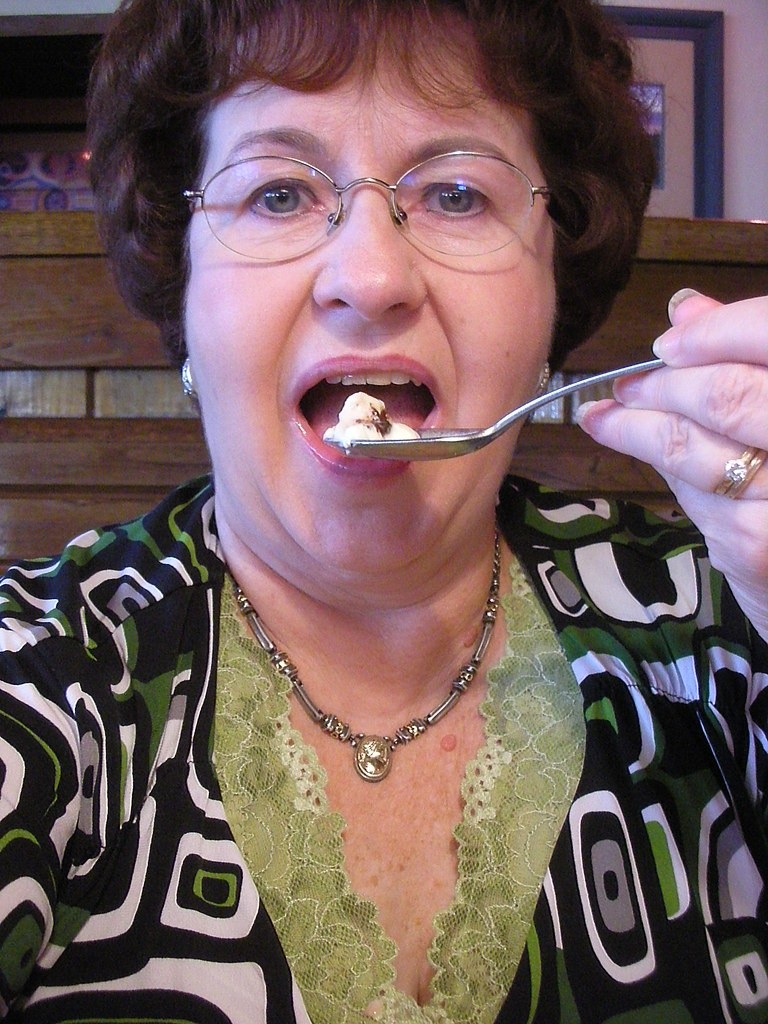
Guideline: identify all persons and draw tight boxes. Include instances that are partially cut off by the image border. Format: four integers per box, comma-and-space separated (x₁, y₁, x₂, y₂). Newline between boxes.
0, 0, 768, 1024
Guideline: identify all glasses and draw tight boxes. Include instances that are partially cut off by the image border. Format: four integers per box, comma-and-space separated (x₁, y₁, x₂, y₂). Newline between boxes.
181, 148, 557, 262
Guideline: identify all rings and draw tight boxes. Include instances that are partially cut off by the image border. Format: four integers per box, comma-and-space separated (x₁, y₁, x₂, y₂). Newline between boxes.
715, 445, 767, 500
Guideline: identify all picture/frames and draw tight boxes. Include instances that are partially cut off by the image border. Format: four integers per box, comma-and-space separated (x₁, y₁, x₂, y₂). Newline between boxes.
595, 4, 724, 223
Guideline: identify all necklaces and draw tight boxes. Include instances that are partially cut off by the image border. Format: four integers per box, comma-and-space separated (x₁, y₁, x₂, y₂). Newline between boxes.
234, 531, 500, 782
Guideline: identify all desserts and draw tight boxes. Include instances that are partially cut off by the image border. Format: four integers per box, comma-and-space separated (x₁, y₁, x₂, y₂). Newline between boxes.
322, 392, 421, 449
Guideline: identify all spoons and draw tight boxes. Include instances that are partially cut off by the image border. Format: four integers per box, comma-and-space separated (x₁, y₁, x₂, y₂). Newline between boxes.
322, 357, 666, 461
